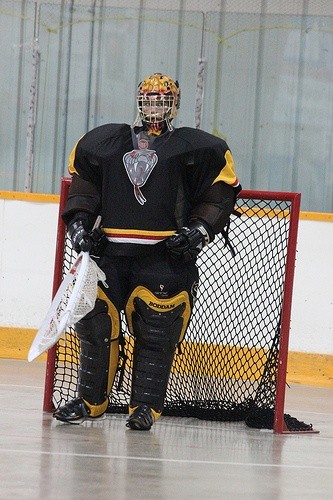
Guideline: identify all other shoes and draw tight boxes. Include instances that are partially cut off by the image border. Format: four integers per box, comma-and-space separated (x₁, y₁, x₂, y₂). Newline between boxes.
53, 397, 106, 425
126, 405, 154, 430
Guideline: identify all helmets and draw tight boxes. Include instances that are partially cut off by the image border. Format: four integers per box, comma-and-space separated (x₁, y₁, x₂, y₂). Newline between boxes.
137, 72, 178, 122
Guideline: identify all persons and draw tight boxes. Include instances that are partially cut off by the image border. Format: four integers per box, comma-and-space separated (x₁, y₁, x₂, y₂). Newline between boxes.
54, 72, 242, 431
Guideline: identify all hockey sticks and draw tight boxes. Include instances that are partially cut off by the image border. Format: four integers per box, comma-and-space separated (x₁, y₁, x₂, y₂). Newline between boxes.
28, 214, 109, 362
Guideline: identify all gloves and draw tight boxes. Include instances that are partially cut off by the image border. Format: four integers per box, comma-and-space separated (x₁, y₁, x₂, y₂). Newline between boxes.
166, 226, 205, 270
66, 216, 108, 259
